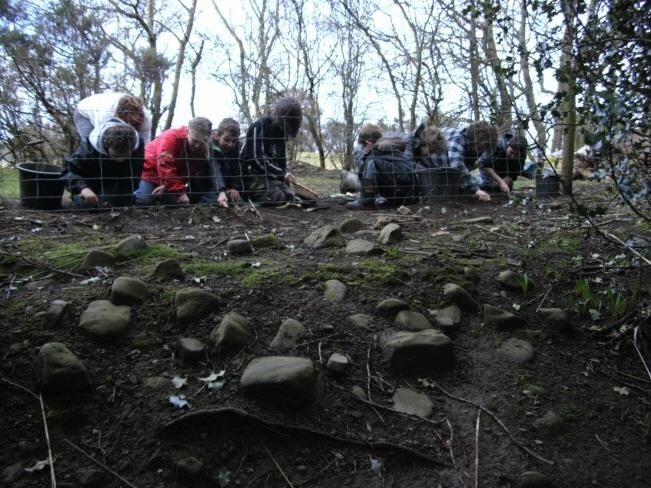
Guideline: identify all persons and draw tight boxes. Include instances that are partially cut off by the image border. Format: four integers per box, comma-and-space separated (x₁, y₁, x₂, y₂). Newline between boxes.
340, 120, 527, 208
63, 91, 303, 207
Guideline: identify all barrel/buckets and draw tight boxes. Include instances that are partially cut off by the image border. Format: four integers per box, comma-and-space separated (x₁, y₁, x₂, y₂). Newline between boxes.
534, 173, 561, 199
15, 161, 67, 210
415, 167, 464, 202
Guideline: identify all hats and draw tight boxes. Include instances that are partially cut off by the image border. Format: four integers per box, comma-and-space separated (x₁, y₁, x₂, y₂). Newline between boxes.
188, 116, 211, 141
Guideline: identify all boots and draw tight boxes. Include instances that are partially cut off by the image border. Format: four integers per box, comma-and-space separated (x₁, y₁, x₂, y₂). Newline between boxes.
347, 178, 376, 210
